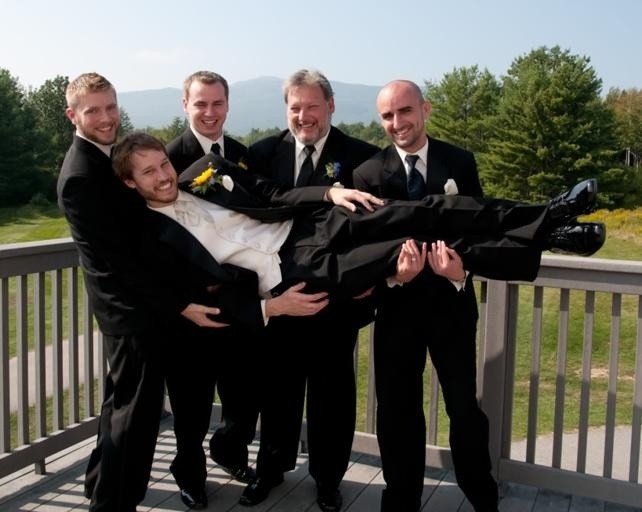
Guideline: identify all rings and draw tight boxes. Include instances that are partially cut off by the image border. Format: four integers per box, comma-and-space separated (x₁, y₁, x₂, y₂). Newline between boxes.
412, 256, 417, 261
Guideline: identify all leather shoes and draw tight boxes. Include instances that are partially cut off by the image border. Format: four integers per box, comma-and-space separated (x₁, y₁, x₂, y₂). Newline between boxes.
181, 486, 207, 509
231, 466, 256, 484
547, 222, 605, 256
551, 179, 598, 217
237, 471, 284, 507
317, 484, 342, 512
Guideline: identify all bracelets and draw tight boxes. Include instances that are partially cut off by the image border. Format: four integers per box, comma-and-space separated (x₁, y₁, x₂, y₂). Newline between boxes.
455, 272, 466, 283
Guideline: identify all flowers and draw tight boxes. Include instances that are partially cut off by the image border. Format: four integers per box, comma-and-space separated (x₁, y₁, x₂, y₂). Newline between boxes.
323, 163, 343, 180
188, 167, 223, 195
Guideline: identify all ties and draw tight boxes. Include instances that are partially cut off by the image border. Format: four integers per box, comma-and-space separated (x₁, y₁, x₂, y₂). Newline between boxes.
296, 146, 316, 186
211, 144, 220, 157
405, 155, 426, 199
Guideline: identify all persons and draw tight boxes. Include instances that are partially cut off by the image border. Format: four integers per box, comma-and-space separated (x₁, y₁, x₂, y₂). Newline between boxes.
237, 67, 398, 510
155, 72, 256, 510
111, 130, 607, 337
56, 72, 231, 510
350, 81, 501, 509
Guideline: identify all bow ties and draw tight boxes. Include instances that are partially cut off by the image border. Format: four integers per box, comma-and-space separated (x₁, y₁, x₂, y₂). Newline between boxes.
175, 202, 199, 226
